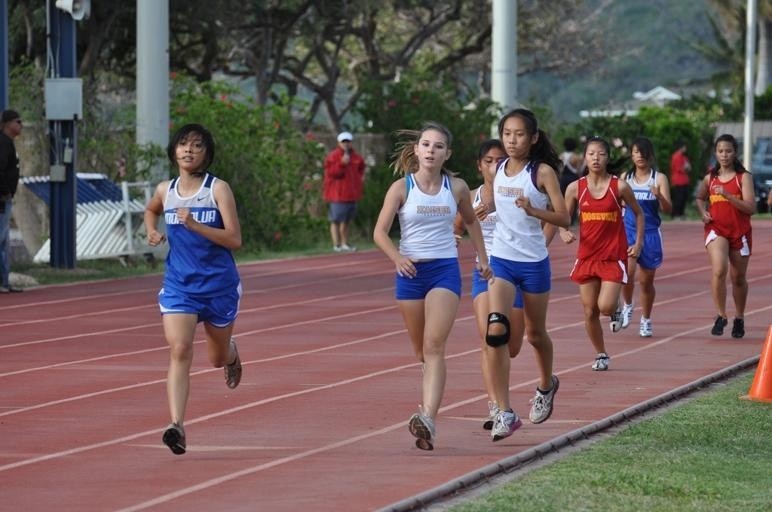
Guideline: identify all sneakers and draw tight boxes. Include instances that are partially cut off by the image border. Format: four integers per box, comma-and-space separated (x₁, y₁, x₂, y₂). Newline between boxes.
711, 314, 727, 335
640, 315, 652, 337
609, 303, 623, 332
408, 413, 436, 450
162, 423, 186, 454
333, 246, 342, 252
622, 304, 632, 328
342, 243, 357, 251
732, 319, 745, 338
483, 401, 499, 430
224, 338, 241, 389
592, 352, 609, 371
491, 409, 523, 441
529, 374, 559, 424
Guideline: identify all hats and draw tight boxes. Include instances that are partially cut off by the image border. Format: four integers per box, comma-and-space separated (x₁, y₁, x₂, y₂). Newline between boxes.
336, 131, 352, 142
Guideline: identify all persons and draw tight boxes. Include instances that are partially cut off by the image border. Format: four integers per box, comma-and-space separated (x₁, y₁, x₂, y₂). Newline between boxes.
556, 138, 582, 227
695, 134, 758, 338
474, 108, 571, 440
557, 138, 645, 370
619, 138, 671, 336
452, 138, 525, 431
143, 122, 242, 454
0, 109, 23, 293
322, 132, 366, 252
372, 120, 493, 451
668, 142, 692, 219
